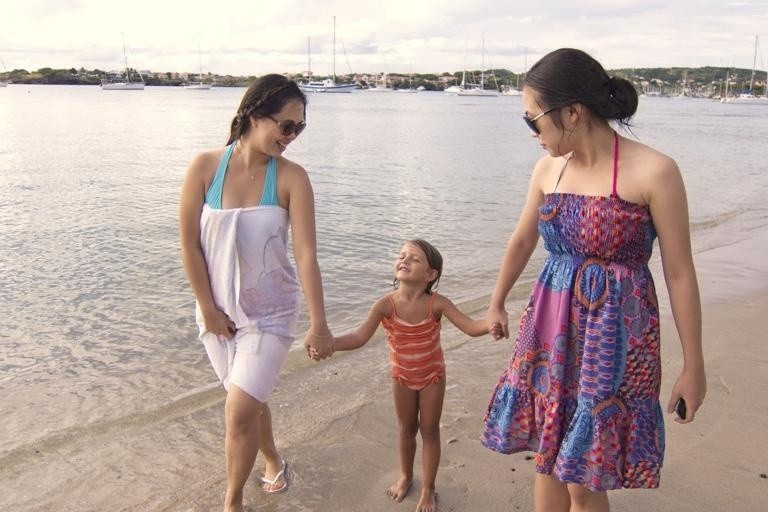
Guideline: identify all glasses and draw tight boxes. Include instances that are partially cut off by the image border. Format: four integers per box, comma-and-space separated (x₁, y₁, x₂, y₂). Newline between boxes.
267, 114, 306, 137
522, 100, 584, 135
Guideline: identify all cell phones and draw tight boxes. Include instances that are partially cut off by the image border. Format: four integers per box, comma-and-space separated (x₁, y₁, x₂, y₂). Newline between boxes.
675, 398, 687, 419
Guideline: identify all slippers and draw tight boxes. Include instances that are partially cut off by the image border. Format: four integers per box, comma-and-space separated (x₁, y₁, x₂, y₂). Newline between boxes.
260, 459, 287, 494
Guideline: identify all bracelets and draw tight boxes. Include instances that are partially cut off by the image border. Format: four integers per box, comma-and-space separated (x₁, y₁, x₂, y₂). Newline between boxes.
313, 330, 333, 340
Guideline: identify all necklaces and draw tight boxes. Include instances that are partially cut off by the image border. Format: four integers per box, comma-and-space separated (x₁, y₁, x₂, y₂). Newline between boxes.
235, 145, 264, 184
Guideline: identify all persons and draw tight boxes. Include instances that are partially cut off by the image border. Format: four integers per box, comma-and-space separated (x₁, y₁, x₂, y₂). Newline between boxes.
311, 237, 503, 511
177, 74, 339, 511
481, 46, 708, 512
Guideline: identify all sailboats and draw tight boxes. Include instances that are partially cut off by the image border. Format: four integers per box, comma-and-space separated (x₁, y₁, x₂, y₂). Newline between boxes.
296, 14, 525, 97
180, 61, 213, 90
641, 32, 766, 105
98, 44, 145, 91
